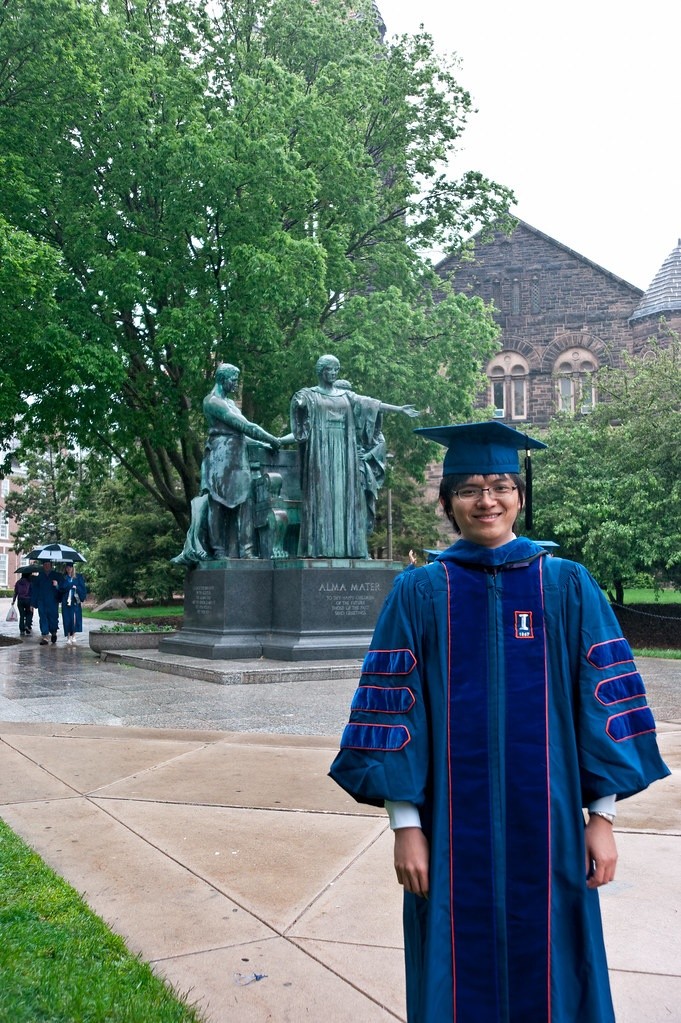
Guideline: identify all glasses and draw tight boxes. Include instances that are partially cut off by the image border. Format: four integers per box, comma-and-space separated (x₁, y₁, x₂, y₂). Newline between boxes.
449, 485, 519, 503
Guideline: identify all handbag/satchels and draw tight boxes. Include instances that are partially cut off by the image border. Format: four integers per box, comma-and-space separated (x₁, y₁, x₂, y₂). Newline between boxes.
6, 606, 18, 622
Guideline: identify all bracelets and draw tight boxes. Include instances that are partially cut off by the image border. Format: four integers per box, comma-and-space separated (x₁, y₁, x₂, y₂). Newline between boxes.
595, 812, 615, 825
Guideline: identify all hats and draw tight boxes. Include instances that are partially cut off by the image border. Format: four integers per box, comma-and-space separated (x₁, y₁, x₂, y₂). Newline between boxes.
65, 562, 77, 567
412, 421, 547, 531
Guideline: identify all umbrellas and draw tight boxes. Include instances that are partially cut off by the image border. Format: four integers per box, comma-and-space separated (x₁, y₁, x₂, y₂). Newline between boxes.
25, 543, 87, 562
14, 565, 42, 573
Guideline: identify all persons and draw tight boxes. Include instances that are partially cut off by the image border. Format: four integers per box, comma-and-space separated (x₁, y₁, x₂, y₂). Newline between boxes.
28, 559, 64, 645
531, 541, 560, 557
11, 573, 33, 635
62, 562, 86, 644
290, 355, 420, 559
327, 421, 672, 1023
393, 549, 443, 587
278, 379, 386, 532
170, 362, 281, 565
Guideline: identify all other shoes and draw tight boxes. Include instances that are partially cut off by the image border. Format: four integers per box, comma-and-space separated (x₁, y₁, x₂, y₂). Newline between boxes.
51, 636, 57, 643
40, 639, 48, 645
71, 635, 76, 642
66, 640, 73, 646
24, 626, 31, 634
20, 631, 24, 634
